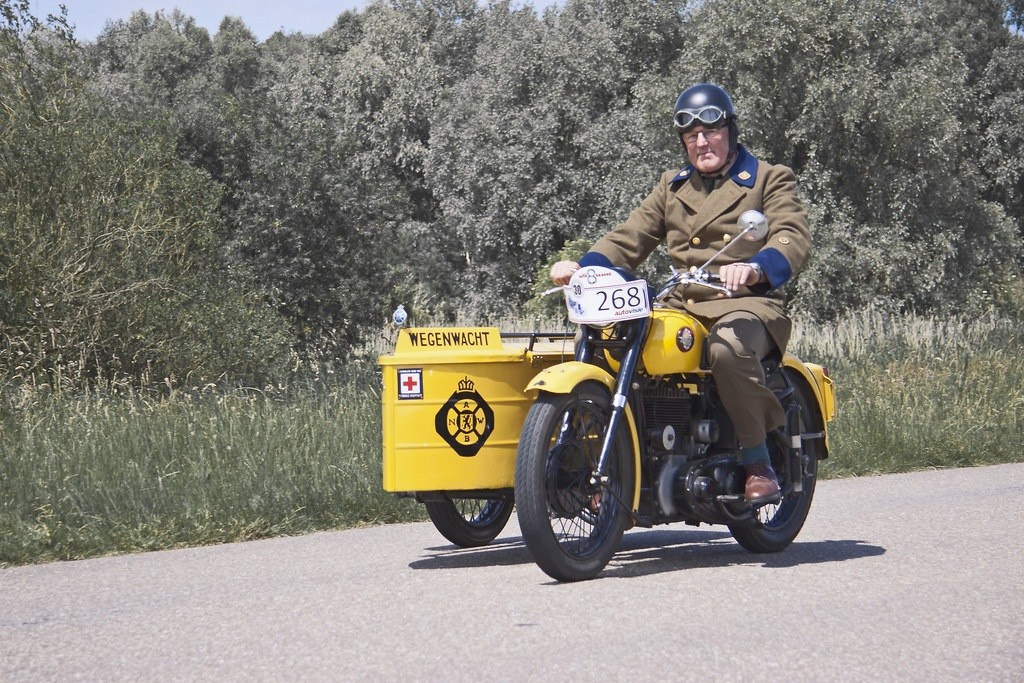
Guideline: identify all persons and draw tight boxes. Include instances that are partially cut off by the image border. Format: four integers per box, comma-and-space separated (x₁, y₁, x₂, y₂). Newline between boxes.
550, 84, 815, 504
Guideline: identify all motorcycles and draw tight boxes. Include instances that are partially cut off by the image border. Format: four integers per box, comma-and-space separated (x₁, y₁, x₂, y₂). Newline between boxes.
382, 209, 837, 582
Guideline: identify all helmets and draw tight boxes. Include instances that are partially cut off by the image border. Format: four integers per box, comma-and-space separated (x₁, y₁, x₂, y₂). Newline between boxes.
673, 83, 735, 127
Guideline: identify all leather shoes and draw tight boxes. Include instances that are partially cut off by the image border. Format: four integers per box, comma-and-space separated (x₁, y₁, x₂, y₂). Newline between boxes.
743, 463, 781, 504
591, 493, 602, 512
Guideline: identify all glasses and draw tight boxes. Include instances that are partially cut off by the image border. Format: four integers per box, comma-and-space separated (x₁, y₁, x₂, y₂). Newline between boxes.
673, 105, 727, 129
